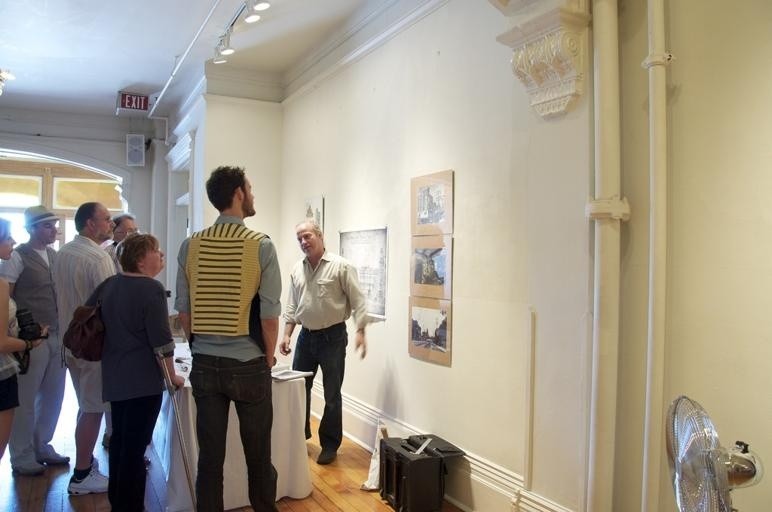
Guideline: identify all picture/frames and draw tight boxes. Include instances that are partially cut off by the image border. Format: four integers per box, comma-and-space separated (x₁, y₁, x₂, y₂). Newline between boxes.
411, 236, 453, 300
408, 297, 452, 367
339, 226, 388, 319
411, 170, 454, 235
303, 195, 325, 238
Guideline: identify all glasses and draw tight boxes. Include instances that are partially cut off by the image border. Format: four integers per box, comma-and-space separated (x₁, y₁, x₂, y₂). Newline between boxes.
117, 231, 134, 236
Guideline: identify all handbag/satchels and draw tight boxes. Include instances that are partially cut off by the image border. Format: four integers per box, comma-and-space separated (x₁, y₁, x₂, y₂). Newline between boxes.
60, 274, 110, 370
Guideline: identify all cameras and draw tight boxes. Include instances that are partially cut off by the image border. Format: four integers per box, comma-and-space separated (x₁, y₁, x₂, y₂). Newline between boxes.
15, 307, 42, 342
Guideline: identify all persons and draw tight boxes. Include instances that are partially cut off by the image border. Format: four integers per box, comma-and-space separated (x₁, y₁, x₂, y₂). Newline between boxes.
1, 205, 71, 475
0, 217, 50, 459
102, 214, 139, 448
51, 203, 116, 497
174, 166, 282, 512
84, 234, 185, 512
279, 217, 368, 463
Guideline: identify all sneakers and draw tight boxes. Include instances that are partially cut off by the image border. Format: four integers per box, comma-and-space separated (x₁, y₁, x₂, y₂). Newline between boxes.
37, 446, 71, 465
66, 468, 109, 496
10, 457, 46, 475
316, 447, 338, 464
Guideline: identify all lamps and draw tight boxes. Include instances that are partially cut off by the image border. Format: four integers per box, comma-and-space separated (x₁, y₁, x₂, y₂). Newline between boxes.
252, 0, 270, 12
245, 2, 261, 23
213, 50, 227, 64
221, 34, 236, 55
0, 67, 14, 101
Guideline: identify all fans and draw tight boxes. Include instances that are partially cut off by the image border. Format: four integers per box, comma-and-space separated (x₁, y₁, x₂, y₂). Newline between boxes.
664, 396, 765, 507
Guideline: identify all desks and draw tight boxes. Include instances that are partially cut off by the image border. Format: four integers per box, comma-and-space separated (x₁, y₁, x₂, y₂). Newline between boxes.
152, 343, 314, 512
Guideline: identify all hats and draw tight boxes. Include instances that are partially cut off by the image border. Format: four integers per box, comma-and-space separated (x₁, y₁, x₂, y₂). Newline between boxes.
24, 205, 61, 228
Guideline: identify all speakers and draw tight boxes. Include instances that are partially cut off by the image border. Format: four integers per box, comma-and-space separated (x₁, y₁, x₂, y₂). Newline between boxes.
125, 133, 146, 167
